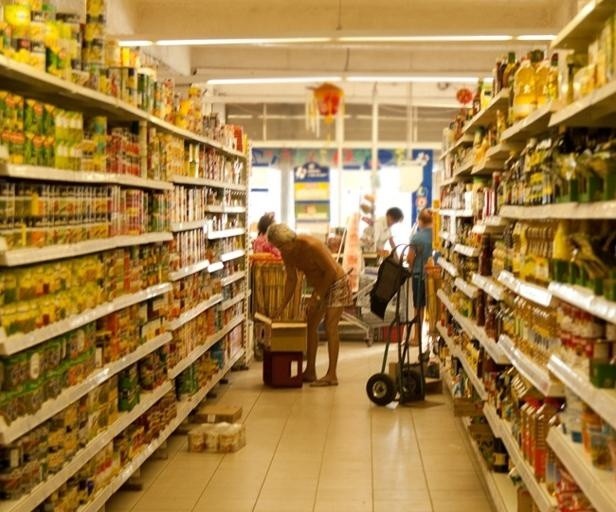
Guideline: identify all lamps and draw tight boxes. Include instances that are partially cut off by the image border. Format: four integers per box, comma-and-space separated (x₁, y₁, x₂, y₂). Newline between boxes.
426, 29, 554, 45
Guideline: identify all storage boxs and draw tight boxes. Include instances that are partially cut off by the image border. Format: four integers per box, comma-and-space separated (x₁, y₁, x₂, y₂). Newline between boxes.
196, 404, 242, 422
187, 422, 246, 453
254, 312, 310, 356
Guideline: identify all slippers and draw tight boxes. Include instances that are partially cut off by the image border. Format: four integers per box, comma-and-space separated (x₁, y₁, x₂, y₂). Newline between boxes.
292, 372, 338, 387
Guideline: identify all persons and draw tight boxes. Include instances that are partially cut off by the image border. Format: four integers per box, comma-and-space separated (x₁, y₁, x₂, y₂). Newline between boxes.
252, 214, 282, 256
376, 206, 413, 269
324, 224, 383, 263
267, 223, 354, 388
402, 209, 433, 346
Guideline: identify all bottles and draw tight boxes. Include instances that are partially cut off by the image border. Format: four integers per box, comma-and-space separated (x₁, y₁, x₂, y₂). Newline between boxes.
517, 396, 564, 496
508, 54, 540, 120
477, 136, 556, 286
474, 287, 616, 392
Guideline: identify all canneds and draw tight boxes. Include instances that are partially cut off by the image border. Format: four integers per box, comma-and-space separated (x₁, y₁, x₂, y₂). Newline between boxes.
189, 422, 245, 452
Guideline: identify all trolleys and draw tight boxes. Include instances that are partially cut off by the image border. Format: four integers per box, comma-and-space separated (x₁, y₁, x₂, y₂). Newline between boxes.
366, 245, 425, 405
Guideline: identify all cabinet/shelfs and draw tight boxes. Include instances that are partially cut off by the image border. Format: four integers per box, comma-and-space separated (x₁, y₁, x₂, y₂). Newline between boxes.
429, 92, 531, 392
162, 121, 250, 406
484, 1, 615, 512
0, 52, 190, 510
